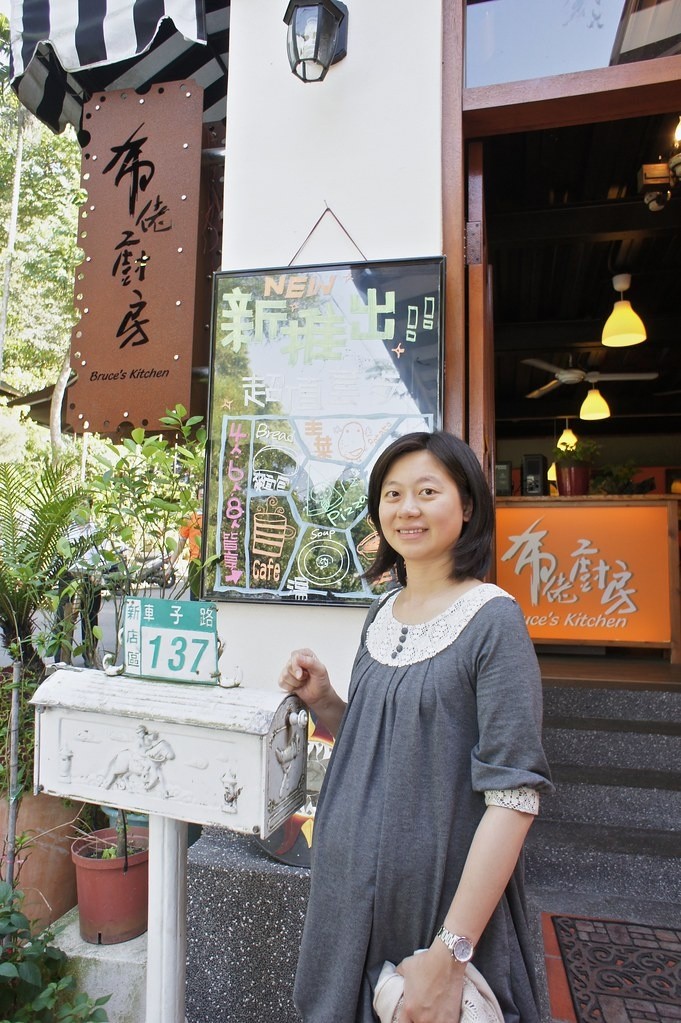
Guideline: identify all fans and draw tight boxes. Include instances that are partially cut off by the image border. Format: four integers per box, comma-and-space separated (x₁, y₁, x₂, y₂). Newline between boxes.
518, 355, 659, 399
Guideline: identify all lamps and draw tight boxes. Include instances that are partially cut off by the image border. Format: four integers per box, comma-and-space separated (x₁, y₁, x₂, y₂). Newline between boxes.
556, 419, 578, 450
580, 383, 611, 420
601, 273, 647, 348
282, 0, 349, 83
547, 459, 557, 480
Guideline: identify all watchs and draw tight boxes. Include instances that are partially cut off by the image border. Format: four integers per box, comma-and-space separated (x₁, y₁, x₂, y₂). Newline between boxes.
437, 927, 475, 962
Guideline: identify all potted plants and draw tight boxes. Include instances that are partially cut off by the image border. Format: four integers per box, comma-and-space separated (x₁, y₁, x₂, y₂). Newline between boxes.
0, 404, 221, 946
552, 438, 601, 496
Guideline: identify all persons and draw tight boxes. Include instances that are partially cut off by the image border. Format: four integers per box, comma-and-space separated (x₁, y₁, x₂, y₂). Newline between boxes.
277, 430, 554, 1023
